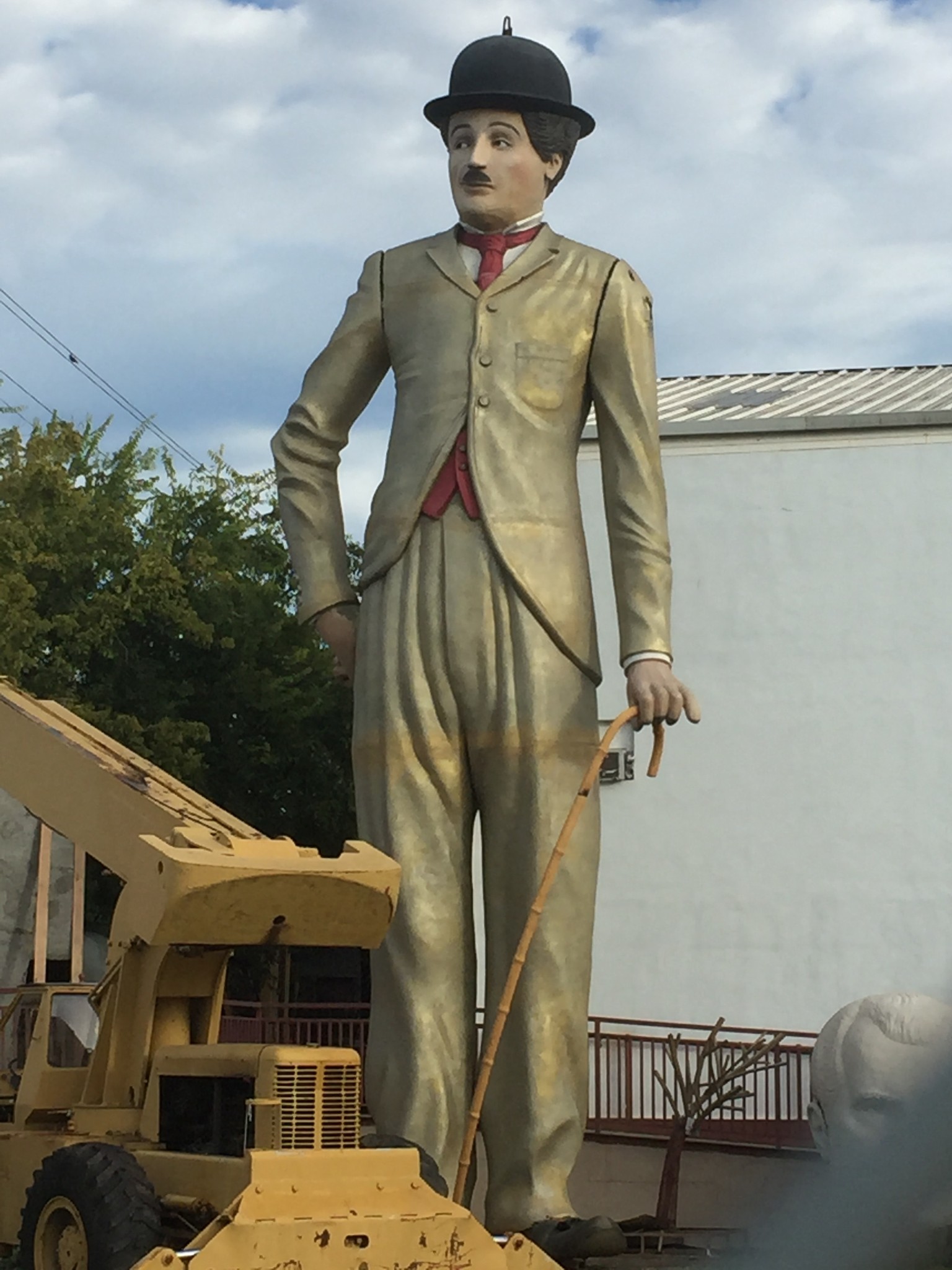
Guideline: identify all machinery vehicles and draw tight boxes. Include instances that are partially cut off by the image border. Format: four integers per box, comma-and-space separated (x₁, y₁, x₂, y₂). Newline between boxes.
0, 672, 568, 1270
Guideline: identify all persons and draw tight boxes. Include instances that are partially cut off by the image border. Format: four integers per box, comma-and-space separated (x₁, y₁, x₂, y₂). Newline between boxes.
807, 994, 951, 1270
270, 15, 702, 1260
